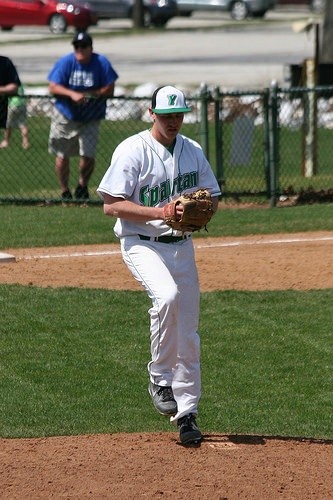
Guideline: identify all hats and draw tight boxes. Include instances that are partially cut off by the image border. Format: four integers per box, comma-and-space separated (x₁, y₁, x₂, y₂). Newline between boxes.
151, 84, 192, 114
72, 32, 92, 44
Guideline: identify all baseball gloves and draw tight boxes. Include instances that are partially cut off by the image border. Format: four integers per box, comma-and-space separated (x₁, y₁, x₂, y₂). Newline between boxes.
164, 189, 213, 233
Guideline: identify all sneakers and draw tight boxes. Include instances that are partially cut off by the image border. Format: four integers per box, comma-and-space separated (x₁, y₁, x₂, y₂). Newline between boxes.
147, 381, 178, 415
176, 413, 203, 445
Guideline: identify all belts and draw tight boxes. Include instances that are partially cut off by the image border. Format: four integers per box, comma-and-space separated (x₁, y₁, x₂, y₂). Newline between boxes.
137, 234, 192, 244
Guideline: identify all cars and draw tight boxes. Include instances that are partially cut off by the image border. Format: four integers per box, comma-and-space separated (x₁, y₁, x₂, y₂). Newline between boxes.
82, 0, 175, 30
177, 1, 271, 22
0, 1, 98, 35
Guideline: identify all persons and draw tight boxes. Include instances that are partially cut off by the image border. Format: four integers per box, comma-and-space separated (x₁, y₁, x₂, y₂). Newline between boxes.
0, 81, 32, 151
0, 56, 21, 130
96, 86, 223, 445
47, 32, 118, 200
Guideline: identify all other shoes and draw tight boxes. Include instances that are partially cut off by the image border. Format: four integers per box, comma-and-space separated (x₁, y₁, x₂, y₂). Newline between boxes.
0, 139, 9, 149
61, 187, 73, 200
21, 140, 31, 150
74, 184, 89, 200
